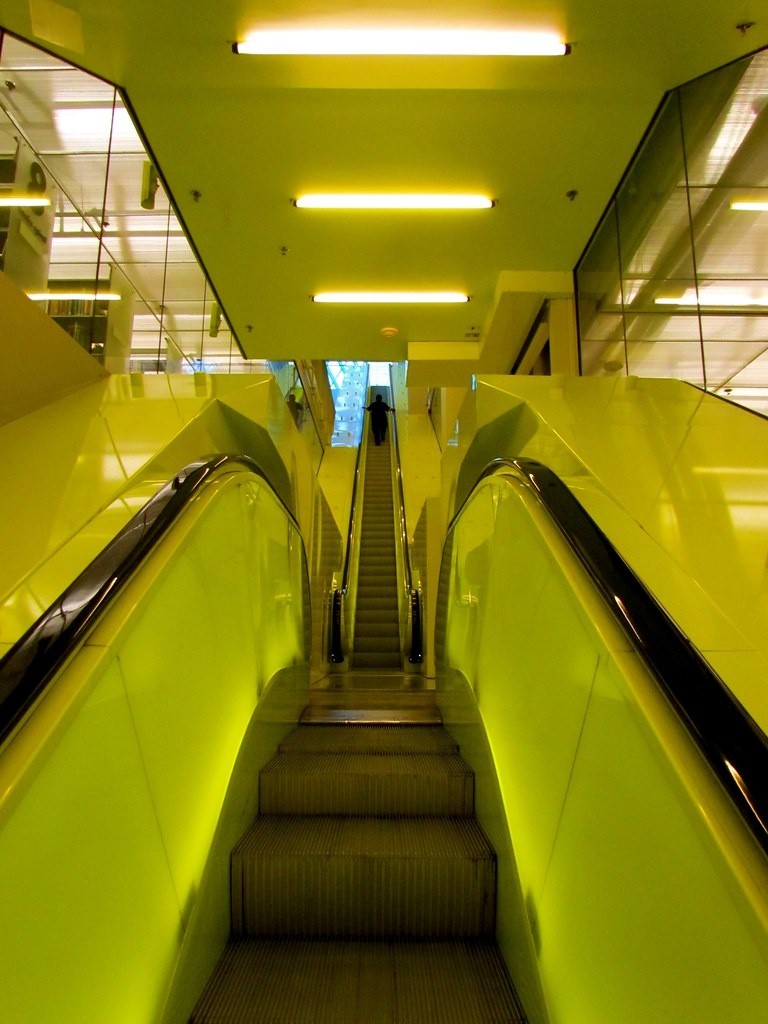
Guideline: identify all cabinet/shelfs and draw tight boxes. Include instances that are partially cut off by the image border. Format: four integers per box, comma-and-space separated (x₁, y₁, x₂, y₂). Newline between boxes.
47, 262, 133, 377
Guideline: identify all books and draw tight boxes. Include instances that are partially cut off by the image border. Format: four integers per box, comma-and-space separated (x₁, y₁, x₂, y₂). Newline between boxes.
48, 294, 107, 356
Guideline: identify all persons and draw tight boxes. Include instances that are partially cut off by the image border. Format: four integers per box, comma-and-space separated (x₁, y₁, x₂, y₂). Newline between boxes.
286, 393, 304, 425
362, 394, 396, 446
